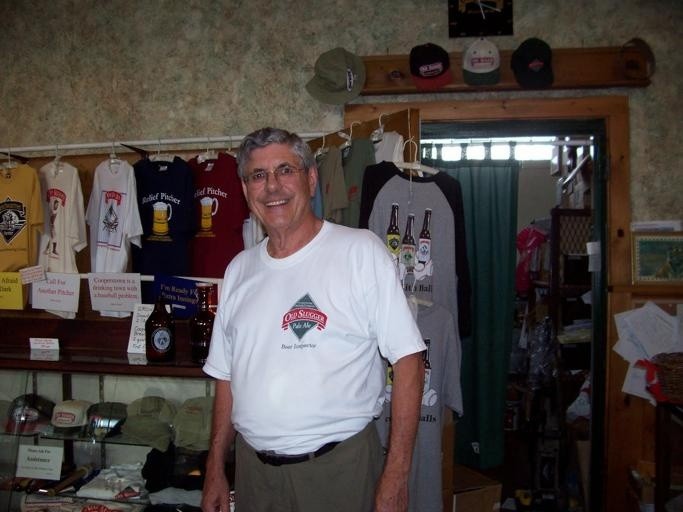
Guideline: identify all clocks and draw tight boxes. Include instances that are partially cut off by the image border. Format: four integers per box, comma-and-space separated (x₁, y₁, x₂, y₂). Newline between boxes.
447, 1, 515, 39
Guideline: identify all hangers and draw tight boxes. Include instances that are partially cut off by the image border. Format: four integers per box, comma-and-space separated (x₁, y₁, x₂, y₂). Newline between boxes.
1, 148, 36, 173
366, 139, 457, 184
339, 120, 371, 151
372, 113, 402, 146
137, 138, 188, 169
94, 140, 133, 169
310, 129, 339, 157
192, 136, 232, 170
40, 146, 79, 175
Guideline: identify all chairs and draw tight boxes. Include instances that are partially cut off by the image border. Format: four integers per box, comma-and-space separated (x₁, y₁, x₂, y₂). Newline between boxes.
529, 205, 592, 330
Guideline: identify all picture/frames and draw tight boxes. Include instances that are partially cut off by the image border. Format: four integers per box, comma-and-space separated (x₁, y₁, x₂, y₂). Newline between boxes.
632, 231, 683, 286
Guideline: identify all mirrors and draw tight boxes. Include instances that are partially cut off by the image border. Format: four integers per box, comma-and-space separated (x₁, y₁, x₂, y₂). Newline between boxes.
419, 115, 606, 512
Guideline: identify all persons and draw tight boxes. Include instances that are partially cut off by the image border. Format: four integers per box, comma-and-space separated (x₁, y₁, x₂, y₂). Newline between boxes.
41, 202, 63, 255
201, 127, 428, 512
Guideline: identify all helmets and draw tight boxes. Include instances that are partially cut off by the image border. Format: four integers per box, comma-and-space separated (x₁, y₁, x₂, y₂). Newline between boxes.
612, 38, 655, 79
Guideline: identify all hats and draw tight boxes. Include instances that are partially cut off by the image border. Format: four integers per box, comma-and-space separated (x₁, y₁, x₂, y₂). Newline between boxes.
51, 400, 93, 435
410, 44, 454, 92
305, 48, 366, 105
80, 402, 128, 441
510, 37, 556, 91
462, 37, 500, 87
6, 394, 55, 433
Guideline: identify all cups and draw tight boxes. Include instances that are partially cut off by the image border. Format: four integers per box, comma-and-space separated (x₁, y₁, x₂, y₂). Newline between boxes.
200, 196, 219, 230
154, 202, 171, 234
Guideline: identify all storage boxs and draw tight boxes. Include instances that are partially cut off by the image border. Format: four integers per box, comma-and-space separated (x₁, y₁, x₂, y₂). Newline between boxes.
453, 463, 501, 511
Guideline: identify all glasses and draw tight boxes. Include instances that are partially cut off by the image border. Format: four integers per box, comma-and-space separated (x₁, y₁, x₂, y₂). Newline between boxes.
244, 166, 310, 183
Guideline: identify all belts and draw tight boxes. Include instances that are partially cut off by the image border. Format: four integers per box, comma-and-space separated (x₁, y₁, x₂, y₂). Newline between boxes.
254, 439, 341, 466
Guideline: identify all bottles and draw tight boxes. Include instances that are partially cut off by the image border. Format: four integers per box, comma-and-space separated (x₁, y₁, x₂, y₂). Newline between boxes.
416, 208, 433, 264
386, 203, 402, 259
400, 212, 416, 273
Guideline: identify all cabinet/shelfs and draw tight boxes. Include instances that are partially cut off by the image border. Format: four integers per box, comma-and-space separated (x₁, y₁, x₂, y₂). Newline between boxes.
0, 307, 239, 512
